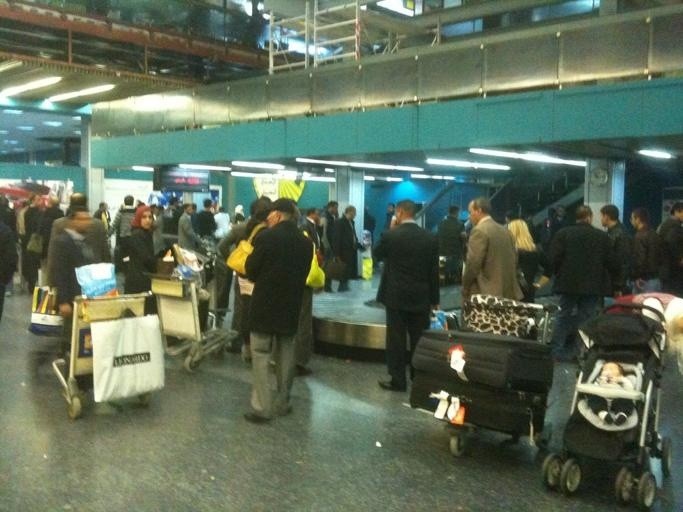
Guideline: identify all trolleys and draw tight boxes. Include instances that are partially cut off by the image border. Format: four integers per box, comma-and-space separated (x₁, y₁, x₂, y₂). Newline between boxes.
143, 271, 239, 373
52, 290, 154, 417
448, 292, 561, 456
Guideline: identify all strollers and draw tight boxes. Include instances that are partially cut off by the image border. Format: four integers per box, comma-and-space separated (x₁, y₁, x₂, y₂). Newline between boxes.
539, 302, 672, 510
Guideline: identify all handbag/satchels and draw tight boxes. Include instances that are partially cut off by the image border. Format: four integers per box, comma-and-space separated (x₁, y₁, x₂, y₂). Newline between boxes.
326, 256, 347, 280
305, 242, 326, 289
429, 310, 459, 330
26, 234, 42, 253
28, 286, 64, 336
89, 308, 166, 406
225, 222, 267, 278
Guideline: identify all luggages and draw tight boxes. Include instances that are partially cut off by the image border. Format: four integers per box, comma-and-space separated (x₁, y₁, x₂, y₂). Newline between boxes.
408, 371, 548, 434
409, 327, 554, 391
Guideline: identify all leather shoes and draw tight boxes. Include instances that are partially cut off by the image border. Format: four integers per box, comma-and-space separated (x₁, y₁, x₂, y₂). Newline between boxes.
376, 378, 408, 392
225, 342, 313, 424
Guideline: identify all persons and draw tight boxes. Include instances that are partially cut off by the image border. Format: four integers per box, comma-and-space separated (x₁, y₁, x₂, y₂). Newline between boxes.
438, 196, 539, 302
299, 201, 366, 292
1, 190, 271, 363
373, 195, 442, 391
588, 356, 635, 424
243, 196, 316, 422
383, 203, 395, 229
538, 202, 681, 361
